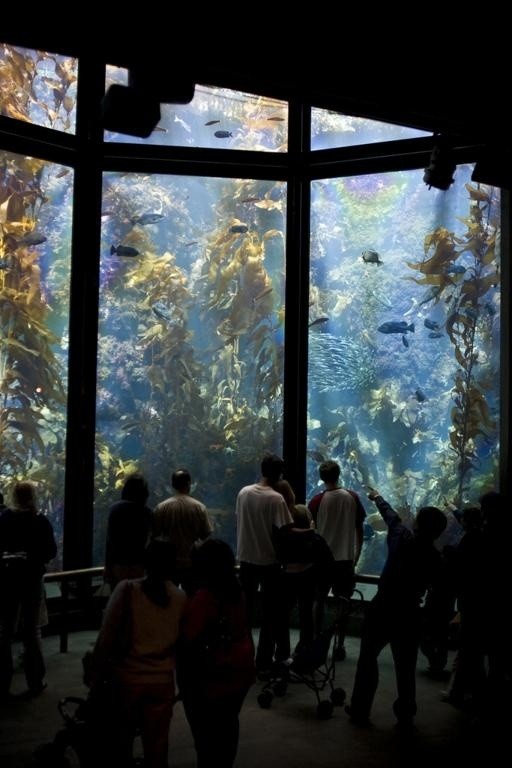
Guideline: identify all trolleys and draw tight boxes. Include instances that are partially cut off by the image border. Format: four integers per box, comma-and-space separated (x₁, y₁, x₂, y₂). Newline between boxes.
255, 588, 365, 719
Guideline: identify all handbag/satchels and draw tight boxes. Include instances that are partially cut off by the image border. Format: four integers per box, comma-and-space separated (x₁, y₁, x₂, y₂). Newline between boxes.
109, 629, 132, 664
